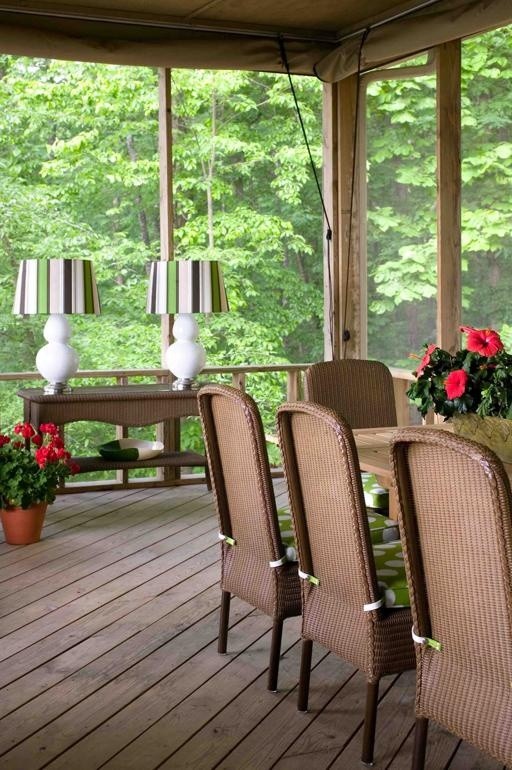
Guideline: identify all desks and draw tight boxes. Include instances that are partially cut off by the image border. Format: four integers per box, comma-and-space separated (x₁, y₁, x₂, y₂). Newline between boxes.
14, 384, 210, 491
267, 421, 454, 523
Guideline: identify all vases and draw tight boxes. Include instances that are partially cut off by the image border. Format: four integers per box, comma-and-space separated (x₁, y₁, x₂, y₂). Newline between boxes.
1, 506, 45, 546
452, 415, 512, 466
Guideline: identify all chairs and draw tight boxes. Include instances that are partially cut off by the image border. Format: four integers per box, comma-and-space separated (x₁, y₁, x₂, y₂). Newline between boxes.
277, 403, 416, 762
388, 426, 512, 770
197, 384, 399, 693
306, 359, 399, 507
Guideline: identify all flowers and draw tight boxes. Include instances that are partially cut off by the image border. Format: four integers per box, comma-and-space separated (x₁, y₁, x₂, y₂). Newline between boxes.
0, 424, 80, 507
407, 324, 512, 422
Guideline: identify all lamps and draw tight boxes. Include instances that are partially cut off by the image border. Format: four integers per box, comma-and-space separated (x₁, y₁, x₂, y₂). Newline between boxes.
147, 259, 230, 391
10, 256, 101, 392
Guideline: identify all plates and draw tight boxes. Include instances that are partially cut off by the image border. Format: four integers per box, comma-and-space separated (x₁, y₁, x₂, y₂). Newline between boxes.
98, 438, 165, 462
